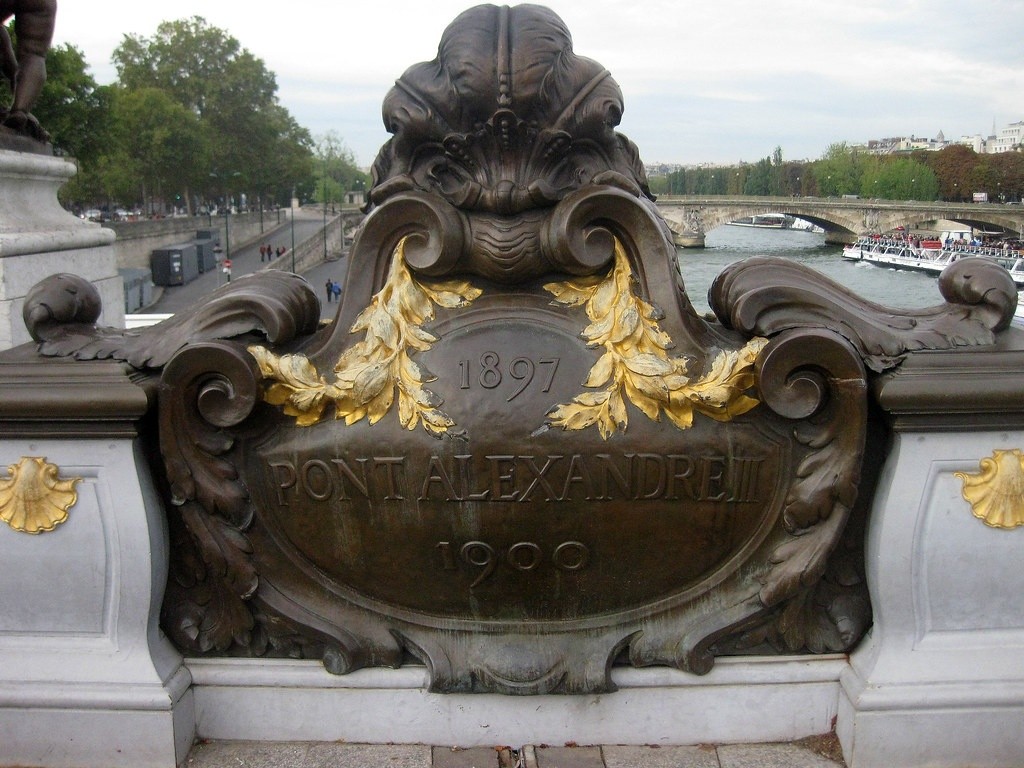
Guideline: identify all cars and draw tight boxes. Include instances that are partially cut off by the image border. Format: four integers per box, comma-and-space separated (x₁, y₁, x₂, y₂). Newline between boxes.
84, 209, 101, 222
1006, 202, 1019, 206
112, 208, 134, 221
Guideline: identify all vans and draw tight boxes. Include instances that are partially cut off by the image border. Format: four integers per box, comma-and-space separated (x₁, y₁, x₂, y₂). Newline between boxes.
841, 195, 861, 200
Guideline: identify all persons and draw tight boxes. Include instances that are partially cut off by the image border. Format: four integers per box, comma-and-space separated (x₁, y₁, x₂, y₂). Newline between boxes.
333, 282, 342, 303
326, 279, 333, 303
793, 193, 801, 197
869, 233, 1022, 259
260, 243, 286, 262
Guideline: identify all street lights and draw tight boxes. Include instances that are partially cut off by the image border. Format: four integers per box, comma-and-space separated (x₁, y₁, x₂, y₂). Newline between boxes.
209, 173, 243, 284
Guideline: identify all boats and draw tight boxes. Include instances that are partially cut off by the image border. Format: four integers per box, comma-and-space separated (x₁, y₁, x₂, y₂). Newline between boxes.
725, 213, 796, 229
841, 230, 1024, 290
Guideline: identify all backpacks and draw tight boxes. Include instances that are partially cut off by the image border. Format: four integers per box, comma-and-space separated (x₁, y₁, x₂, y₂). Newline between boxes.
327, 283, 332, 289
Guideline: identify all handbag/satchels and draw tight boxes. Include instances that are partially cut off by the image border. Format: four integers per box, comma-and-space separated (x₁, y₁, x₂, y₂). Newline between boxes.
338, 287, 341, 293
269, 251, 273, 254
